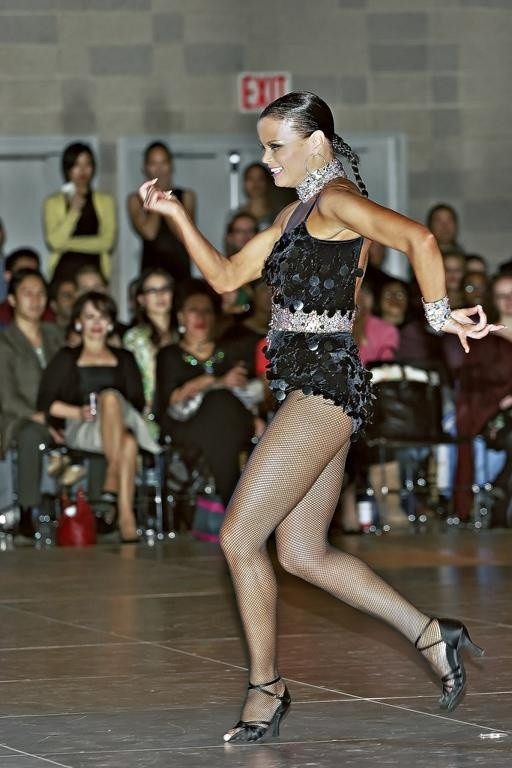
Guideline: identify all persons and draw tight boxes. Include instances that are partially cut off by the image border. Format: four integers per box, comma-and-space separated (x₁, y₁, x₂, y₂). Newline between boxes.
134, 91, 512, 742
1, 141, 511, 550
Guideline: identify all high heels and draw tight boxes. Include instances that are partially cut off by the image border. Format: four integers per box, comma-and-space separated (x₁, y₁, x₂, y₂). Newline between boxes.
414, 617, 485, 712
223, 675, 291, 744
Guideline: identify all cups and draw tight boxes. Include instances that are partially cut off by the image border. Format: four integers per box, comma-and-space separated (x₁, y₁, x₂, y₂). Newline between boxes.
60, 180, 76, 201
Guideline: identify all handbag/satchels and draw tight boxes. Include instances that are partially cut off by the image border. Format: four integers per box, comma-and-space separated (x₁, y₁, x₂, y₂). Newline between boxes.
191, 478, 225, 542
366, 345, 442, 441
55, 489, 98, 545
